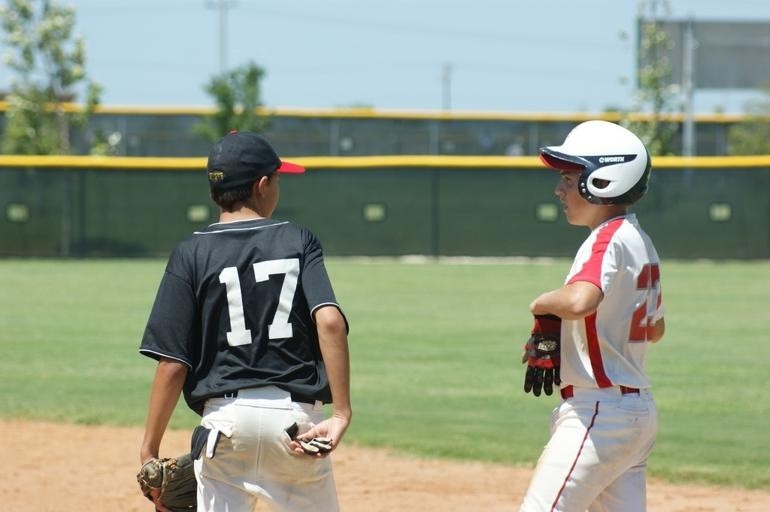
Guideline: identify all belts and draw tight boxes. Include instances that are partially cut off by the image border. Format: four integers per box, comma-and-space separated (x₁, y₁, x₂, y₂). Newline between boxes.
209, 389, 317, 406
559, 383, 641, 400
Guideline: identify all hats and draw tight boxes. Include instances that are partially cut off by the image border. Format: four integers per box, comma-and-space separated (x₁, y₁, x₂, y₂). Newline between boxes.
204, 128, 309, 191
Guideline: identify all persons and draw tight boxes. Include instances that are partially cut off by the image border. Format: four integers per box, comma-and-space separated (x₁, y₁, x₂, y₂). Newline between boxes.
135, 128, 353, 512
515, 118, 666, 512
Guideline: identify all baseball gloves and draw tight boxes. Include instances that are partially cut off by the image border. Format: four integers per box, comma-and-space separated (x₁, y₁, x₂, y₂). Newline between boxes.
139, 454, 197, 512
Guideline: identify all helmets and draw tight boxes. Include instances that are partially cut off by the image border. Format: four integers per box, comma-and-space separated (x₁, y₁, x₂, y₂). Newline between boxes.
536, 118, 654, 208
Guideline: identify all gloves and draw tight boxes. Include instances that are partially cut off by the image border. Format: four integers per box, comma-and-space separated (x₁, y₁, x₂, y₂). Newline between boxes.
286, 421, 334, 459
520, 312, 563, 398
189, 425, 235, 463
